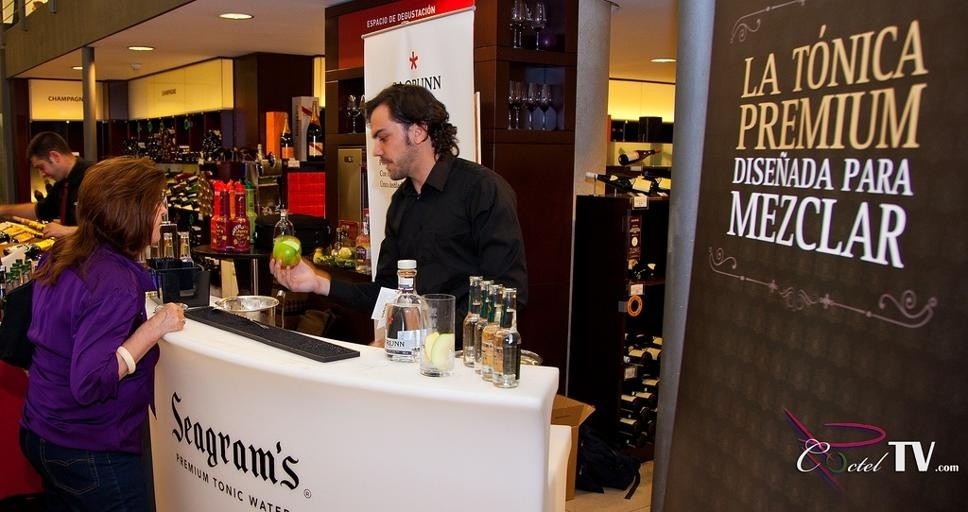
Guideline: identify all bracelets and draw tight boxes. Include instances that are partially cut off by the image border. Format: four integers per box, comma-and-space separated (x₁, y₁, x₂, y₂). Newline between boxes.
116, 346, 137, 374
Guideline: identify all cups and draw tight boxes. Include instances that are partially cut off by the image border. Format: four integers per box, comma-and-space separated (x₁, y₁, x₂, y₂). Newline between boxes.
420, 294, 457, 378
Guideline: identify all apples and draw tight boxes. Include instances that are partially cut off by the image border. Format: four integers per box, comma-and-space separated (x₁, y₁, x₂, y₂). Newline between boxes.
272, 234, 303, 268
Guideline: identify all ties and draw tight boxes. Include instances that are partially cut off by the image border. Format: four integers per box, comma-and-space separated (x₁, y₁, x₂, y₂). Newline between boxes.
60, 181, 68, 225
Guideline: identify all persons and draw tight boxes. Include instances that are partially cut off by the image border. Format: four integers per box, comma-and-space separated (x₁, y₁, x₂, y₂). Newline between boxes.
0, 131, 96, 240
19, 155, 186, 512
268, 82, 529, 353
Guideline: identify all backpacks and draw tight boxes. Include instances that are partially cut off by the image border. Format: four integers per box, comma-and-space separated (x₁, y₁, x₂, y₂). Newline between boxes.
580, 426, 641, 499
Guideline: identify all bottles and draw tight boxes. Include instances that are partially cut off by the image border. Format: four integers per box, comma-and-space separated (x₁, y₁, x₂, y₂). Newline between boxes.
136, 171, 257, 268
463, 275, 521, 388
620, 332, 662, 449
330, 207, 371, 276
280, 113, 295, 165
124, 114, 274, 177
384, 260, 421, 364
511, 0, 548, 52
508, 80, 552, 131
629, 262, 657, 281
585, 147, 671, 195
345, 94, 364, 134
0, 215, 55, 324
273, 209, 295, 250
307, 101, 324, 162
